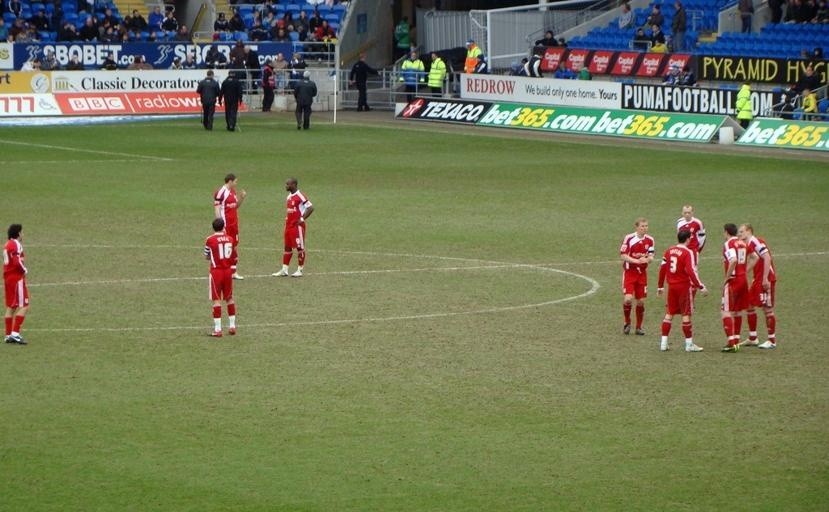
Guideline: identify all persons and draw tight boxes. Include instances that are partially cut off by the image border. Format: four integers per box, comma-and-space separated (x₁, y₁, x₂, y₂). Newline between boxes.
635, 26, 669, 54
261, 58, 278, 113
428, 53, 447, 98
510, 48, 544, 78
1, 223, 32, 346
272, 176, 315, 278
348, 53, 382, 113
203, 217, 238, 337
77, 5, 193, 43
213, 173, 246, 280
781, 47, 826, 119
619, 4, 637, 29
619, 218, 656, 335
129, 54, 154, 70
272, 52, 308, 93
737, 223, 777, 349
292, 70, 318, 130
206, 41, 262, 93
719, 222, 760, 353
100, 53, 119, 70
672, 2, 687, 50
645, 4, 664, 28
399, 52, 426, 103
536, 30, 567, 48
767, 1, 829, 26
556, 61, 578, 79
21, 48, 88, 72
197, 70, 221, 132
738, 0, 754, 35
213, 5, 338, 42
735, 81, 754, 128
222, 70, 243, 132
463, 39, 486, 74
663, 65, 697, 88
1, 0, 79, 43
170, 54, 198, 70
656, 228, 708, 353
675, 204, 707, 312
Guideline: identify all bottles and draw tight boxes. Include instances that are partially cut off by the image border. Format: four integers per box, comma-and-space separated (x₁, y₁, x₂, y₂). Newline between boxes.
466, 39, 474, 45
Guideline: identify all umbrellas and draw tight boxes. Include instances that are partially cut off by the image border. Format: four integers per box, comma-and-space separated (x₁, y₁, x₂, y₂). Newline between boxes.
204, 125, 207, 130
229, 127, 234, 131
304, 127, 310, 130
226, 126, 229, 130
208, 127, 212, 130
297, 125, 302, 129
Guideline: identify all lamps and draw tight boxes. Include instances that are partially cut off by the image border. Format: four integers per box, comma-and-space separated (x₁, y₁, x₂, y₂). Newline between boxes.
688, 23, 828, 60
238, 4, 345, 31
0, 1, 123, 40
565, 1, 738, 54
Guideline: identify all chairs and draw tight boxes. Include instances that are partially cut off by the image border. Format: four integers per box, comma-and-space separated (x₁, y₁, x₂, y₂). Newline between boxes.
232, 274, 244, 280
4, 337, 11, 343
685, 344, 703, 352
229, 327, 235, 335
623, 325, 630, 335
661, 343, 669, 351
8, 335, 26, 344
740, 339, 759, 346
636, 329, 644, 334
209, 331, 222, 337
735, 344, 740, 351
721, 345, 737, 353
291, 271, 303, 277
758, 341, 776, 349
272, 270, 289, 276
